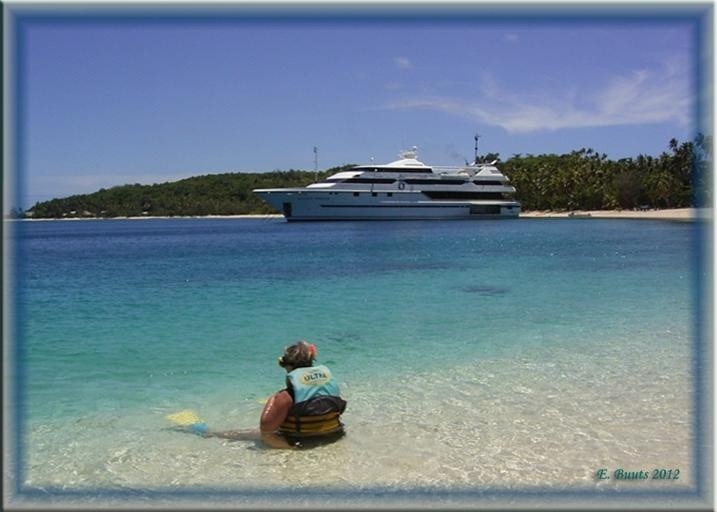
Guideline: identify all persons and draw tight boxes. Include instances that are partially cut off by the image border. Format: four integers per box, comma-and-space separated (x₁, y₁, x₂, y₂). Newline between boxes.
167, 341, 348, 450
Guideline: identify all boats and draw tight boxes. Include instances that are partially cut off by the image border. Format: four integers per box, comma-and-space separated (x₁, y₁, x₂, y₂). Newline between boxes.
251, 147, 523, 224
